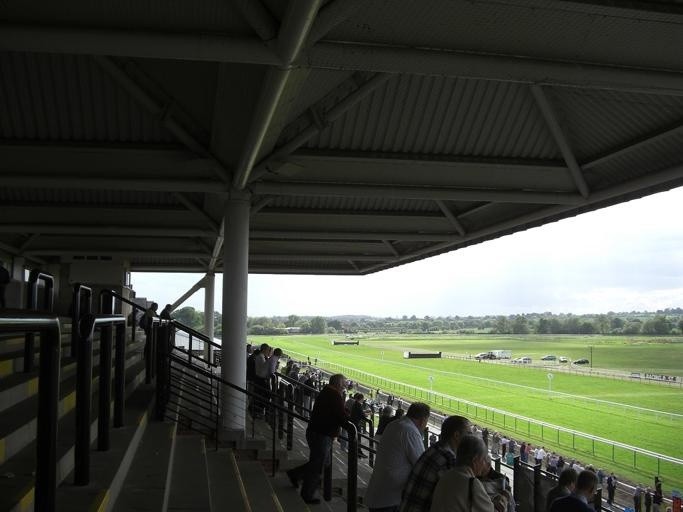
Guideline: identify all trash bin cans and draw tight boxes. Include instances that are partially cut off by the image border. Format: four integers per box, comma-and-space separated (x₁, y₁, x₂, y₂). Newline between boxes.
506, 453, 513, 464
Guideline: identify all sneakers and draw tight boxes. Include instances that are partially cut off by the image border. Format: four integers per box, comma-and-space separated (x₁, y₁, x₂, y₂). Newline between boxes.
303, 496, 320, 504
358, 451, 367, 458
286, 469, 299, 488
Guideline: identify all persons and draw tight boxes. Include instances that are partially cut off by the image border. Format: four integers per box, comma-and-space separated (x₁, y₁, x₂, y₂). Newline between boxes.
139, 301, 158, 360
286, 374, 405, 505
127, 309, 140, 327
360, 402, 666, 511
161, 304, 174, 320
246, 343, 325, 423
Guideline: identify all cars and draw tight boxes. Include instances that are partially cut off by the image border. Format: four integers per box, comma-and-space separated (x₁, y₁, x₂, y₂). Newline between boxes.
511, 356, 533, 363
573, 359, 589, 364
541, 355, 557, 360
474, 353, 489, 359
559, 356, 568, 362
